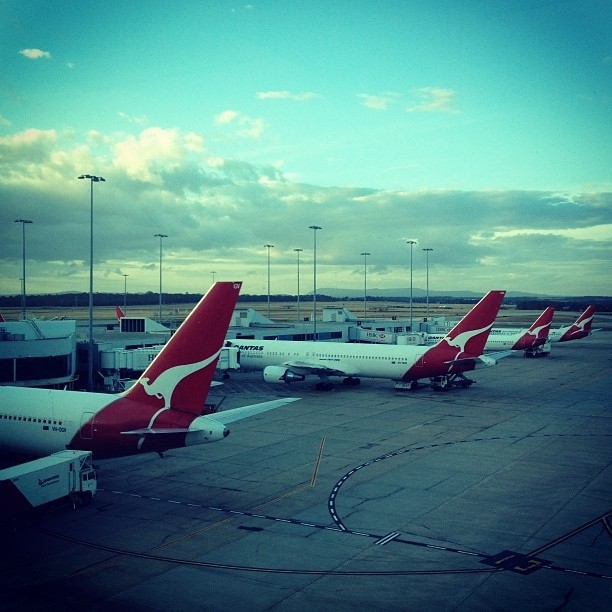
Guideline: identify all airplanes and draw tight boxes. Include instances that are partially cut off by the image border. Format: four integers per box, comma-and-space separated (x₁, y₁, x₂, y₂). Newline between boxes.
423, 304, 555, 360
490, 302, 596, 343
0, 281, 243, 468
225, 290, 507, 398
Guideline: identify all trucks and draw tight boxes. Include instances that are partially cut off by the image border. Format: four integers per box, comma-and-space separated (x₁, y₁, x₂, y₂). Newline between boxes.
0, 449, 97, 514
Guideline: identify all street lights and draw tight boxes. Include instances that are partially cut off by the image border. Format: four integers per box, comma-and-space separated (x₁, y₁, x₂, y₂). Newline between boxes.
294, 248, 304, 322
405, 238, 419, 333
263, 244, 275, 320
152, 234, 169, 328
13, 218, 34, 318
308, 225, 321, 341
77, 174, 106, 390
422, 248, 434, 317
360, 252, 371, 323
